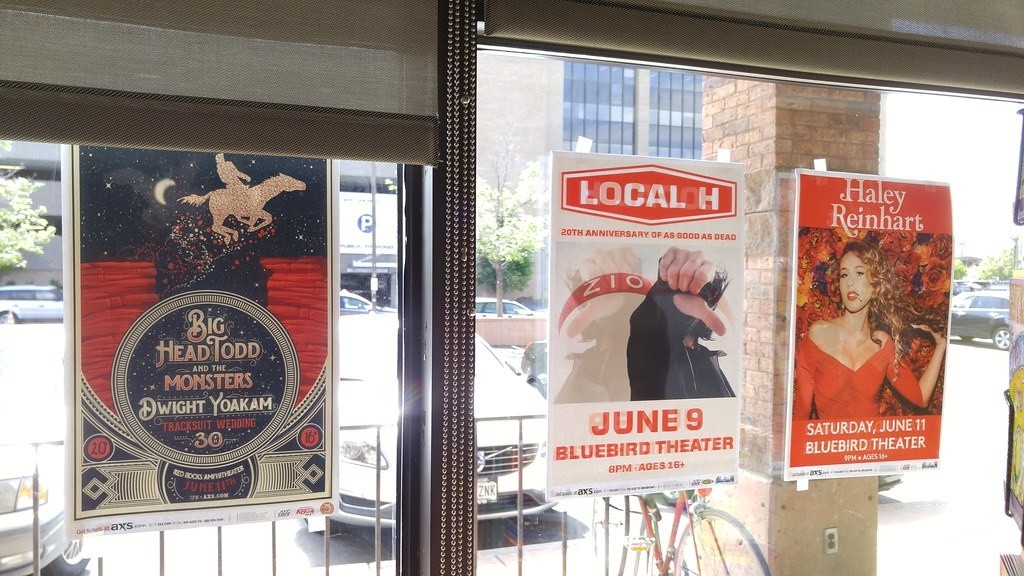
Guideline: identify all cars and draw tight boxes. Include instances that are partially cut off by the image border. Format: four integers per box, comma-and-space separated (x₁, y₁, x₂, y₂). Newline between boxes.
0, 283, 95, 576
336, 289, 560, 528
949, 279, 1012, 351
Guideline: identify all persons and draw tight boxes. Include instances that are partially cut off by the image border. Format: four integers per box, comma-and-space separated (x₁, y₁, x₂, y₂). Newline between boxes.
627, 246, 736, 402
793, 235, 946, 421
